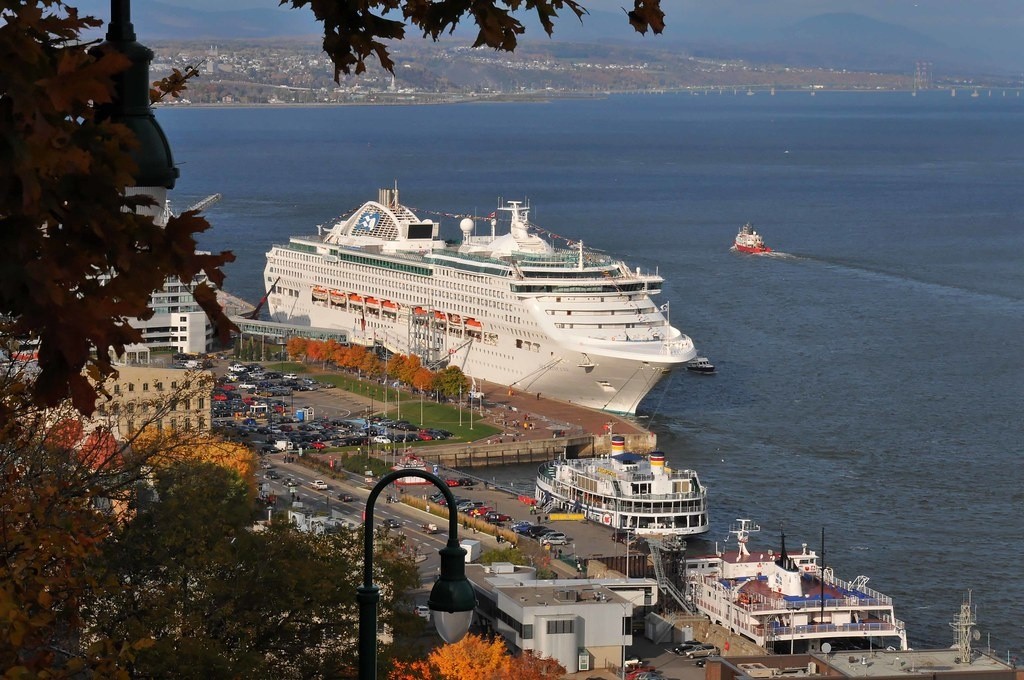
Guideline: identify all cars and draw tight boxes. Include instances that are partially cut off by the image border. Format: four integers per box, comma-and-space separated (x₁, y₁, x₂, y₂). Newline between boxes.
414, 605, 429, 618
213, 386, 454, 454
382, 519, 399, 528
430, 490, 567, 546
173, 353, 228, 369
260, 460, 272, 469
263, 471, 280, 479
219, 360, 336, 397
419, 523, 439, 534
444, 478, 472, 487
282, 477, 299, 487
337, 492, 353, 502
674, 641, 720, 668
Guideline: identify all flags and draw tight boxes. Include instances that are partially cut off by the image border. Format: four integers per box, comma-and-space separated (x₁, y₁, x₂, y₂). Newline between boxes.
321, 203, 659, 337
660, 303, 668, 311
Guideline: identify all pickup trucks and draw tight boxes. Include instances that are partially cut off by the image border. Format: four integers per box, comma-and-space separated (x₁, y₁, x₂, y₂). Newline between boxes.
310, 479, 327, 490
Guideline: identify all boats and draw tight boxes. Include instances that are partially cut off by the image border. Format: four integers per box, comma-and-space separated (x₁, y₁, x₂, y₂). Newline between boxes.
686, 356, 714, 375
735, 223, 771, 253
535, 420, 710, 535
678, 517, 912, 656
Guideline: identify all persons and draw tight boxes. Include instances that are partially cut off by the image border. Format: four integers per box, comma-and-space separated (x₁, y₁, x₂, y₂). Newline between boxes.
283, 393, 583, 574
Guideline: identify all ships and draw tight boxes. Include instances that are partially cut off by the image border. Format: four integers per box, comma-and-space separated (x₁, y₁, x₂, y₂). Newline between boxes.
264, 178, 696, 417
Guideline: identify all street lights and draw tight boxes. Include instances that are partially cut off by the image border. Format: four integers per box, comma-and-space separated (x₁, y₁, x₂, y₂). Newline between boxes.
356, 468, 477, 680
